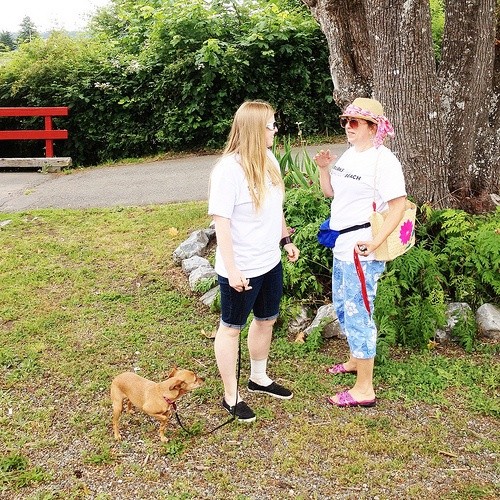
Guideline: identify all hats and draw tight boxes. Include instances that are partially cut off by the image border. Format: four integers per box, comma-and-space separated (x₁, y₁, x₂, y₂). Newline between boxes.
339, 98, 396, 150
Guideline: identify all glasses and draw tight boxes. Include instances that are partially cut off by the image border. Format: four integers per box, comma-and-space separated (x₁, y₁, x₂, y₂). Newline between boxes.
339, 118, 373, 129
266, 121, 279, 131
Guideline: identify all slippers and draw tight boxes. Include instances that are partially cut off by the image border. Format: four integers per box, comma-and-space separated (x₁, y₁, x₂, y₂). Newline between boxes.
328, 389, 376, 407
326, 363, 357, 374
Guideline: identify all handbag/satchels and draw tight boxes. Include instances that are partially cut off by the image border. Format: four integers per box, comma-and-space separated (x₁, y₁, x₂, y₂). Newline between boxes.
316, 219, 339, 248
371, 200, 417, 262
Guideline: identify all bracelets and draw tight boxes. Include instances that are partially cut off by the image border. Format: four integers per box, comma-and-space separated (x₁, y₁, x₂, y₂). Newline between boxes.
280, 236, 293, 249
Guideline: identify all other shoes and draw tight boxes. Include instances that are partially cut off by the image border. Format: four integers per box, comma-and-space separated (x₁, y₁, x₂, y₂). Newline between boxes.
247, 378, 293, 399
222, 397, 256, 423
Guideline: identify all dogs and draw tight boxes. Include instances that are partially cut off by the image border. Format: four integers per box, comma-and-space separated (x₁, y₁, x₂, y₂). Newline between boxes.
110, 365, 206, 443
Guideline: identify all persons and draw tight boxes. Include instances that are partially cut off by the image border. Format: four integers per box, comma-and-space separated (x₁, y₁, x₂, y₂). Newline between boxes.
208, 101, 301, 423
314, 98, 407, 407
